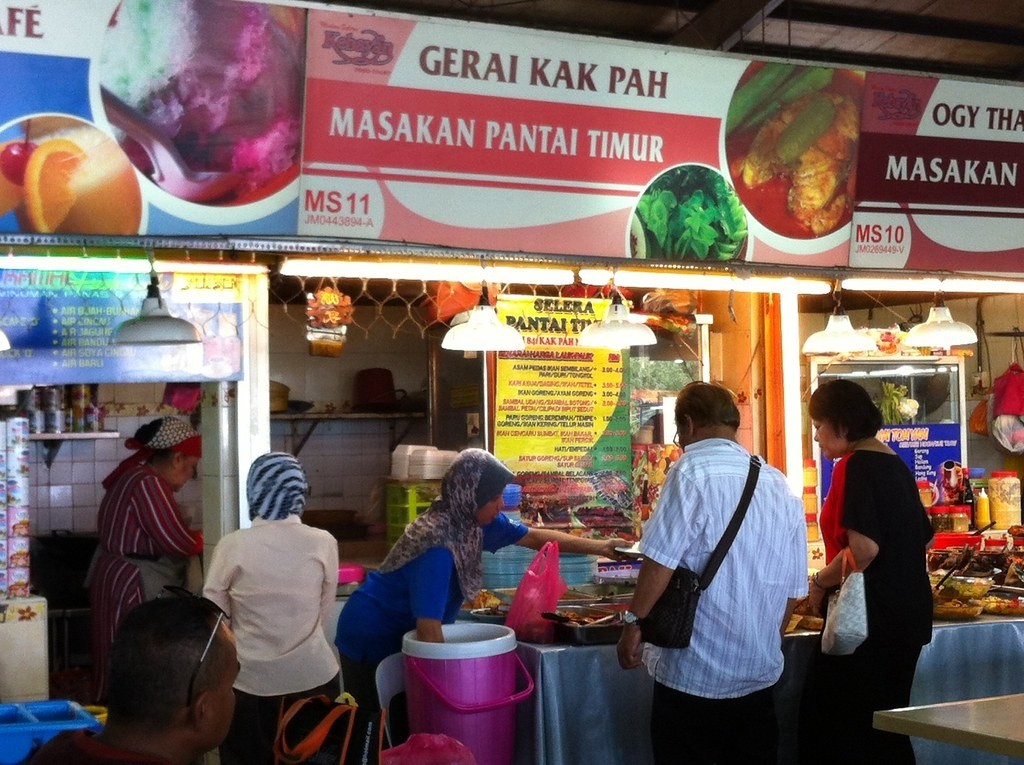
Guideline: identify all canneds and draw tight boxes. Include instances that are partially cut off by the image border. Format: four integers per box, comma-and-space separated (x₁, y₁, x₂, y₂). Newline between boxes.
21, 383, 106, 434
0, 417, 31, 600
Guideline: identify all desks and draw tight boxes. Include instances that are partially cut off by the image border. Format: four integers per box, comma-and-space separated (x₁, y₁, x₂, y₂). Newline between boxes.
872, 689, 1023, 764
515, 615, 1023, 765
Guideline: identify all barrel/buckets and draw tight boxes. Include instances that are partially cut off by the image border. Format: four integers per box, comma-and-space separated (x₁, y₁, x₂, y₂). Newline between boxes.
352, 369, 406, 413
558, 552, 599, 585
933, 533, 981, 551
402, 624, 535, 765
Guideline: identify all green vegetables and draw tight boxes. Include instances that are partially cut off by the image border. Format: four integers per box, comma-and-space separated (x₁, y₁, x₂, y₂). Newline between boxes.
635, 165, 748, 262
875, 380, 909, 425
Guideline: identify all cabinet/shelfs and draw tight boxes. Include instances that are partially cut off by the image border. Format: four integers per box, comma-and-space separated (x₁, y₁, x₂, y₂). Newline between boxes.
387, 479, 443, 553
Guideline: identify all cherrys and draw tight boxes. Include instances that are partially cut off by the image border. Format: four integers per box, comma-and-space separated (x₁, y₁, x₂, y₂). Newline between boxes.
0, 118, 41, 186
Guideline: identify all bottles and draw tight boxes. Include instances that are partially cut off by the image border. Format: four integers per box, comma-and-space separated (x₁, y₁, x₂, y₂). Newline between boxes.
479, 484, 543, 604
989, 472, 1021, 529
929, 547, 1004, 567
803, 457, 819, 542
1013, 538, 1024, 552
984, 540, 1007, 553
927, 506, 969, 532
959, 467, 991, 530
916, 481, 931, 507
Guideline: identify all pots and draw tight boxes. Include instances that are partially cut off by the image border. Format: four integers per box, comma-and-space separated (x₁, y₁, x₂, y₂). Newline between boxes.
35, 530, 100, 604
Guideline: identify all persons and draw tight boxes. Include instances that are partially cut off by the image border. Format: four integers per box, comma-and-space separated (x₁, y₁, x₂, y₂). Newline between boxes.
802, 379, 934, 765
334, 448, 634, 765
85, 417, 203, 703
203, 450, 340, 765
616, 383, 808, 765
25, 583, 239, 765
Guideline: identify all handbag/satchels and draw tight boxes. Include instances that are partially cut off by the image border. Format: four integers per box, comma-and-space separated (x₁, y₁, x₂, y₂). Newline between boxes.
821, 545, 868, 656
502, 541, 566, 641
273, 693, 388, 765
969, 362, 1024, 455
642, 565, 702, 649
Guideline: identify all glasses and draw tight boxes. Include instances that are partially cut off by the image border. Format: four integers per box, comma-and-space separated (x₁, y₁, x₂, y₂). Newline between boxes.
155, 584, 230, 706
673, 429, 683, 449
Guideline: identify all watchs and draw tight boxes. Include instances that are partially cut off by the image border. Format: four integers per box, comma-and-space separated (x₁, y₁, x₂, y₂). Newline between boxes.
623, 611, 640, 625
812, 572, 821, 586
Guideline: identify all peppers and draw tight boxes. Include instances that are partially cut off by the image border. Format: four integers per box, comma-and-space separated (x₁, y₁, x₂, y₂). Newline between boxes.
723, 62, 842, 166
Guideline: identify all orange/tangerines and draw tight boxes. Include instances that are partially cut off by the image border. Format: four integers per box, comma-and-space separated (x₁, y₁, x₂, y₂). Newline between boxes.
23, 138, 85, 236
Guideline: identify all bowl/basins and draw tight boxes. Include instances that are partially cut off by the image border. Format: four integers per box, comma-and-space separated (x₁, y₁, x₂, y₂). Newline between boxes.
626, 60, 852, 262
933, 598, 986, 618
594, 569, 640, 586
933, 567, 1002, 580
0, 1, 303, 239
928, 573, 995, 599
983, 592, 1024, 616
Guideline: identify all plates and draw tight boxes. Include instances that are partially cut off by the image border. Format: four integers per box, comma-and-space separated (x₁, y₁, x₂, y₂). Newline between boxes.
615, 542, 644, 558
470, 607, 510, 623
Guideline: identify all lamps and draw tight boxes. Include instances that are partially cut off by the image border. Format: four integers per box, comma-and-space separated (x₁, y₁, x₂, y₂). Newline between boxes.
441, 281, 525, 351
802, 291, 978, 354
578, 297, 658, 348
110, 252, 202, 344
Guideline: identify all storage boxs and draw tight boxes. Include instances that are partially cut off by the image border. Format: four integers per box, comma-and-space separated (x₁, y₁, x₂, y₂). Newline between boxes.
0, 699, 102, 765
